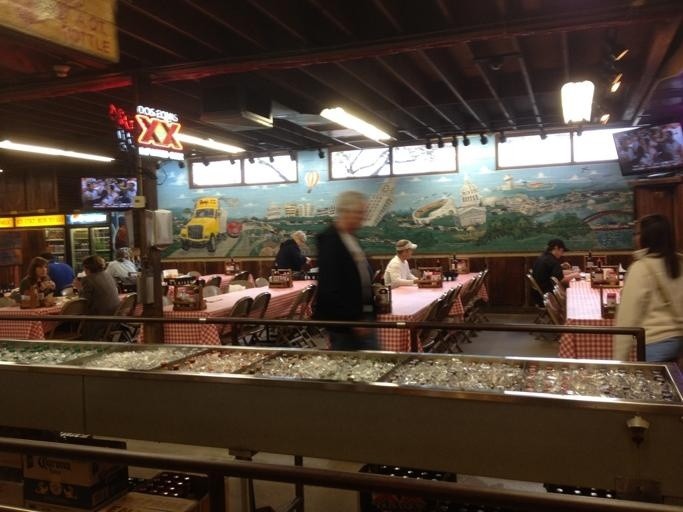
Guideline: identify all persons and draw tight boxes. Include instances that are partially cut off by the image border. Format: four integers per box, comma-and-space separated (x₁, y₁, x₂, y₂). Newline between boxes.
132, 247, 142, 271
19, 257, 56, 298
115, 224, 128, 249
618, 123, 683, 173
311, 192, 381, 351
105, 248, 138, 284
81, 177, 138, 208
42, 253, 75, 296
612, 213, 683, 361
128, 248, 134, 261
72, 255, 120, 341
529, 239, 582, 308
274, 230, 311, 271
382, 239, 439, 348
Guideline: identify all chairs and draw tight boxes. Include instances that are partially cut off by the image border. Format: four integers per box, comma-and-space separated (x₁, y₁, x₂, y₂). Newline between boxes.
551, 275, 565, 295
129, 271, 143, 281
203, 286, 221, 299
56, 283, 74, 297
103, 295, 137, 345
193, 278, 205, 290
46, 297, 88, 342
441, 288, 463, 352
8, 287, 20, 302
220, 296, 253, 347
553, 285, 566, 309
451, 284, 472, 343
255, 277, 269, 288
543, 291, 565, 336
207, 277, 222, 294
164, 276, 196, 300
272, 284, 317, 347
526, 274, 549, 303
233, 271, 249, 282
461, 276, 478, 336
478, 268, 490, 320
251, 291, 272, 348
226, 279, 248, 292
410, 298, 451, 354
528, 268, 533, 274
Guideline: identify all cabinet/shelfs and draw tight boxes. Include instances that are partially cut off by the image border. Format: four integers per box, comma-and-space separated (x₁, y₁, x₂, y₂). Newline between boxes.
70, 227, 111, 279
41, 229, 66, 267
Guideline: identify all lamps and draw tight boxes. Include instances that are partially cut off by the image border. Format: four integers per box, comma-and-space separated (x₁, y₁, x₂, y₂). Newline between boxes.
247, 154, 254, 164
598, 80, 622, 96
608, 40, 629, 62
319, 145, 326, 158
561, 80, 596, 136
452, 136, 459, 148
600, 67, 623, 84
479, 134, 487, 145
268, 152, 276, 163
499, 130, 507, 143
540, 126, 547, 141
204, 157, 210, 166
288, 149, 296, 161
229, 157, 235, 165
462, 135, 471, 148
425, 137, 433, 149
178, 160, 185, 169
438, 135, 444, 147
592, 102, 610, 124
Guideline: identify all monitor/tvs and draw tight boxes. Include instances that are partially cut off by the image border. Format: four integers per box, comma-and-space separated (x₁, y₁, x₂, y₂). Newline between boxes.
78, 175, 140, 212
611, 120, 683, 177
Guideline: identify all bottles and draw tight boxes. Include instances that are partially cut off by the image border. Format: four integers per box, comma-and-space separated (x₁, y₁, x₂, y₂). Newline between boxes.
274, 264, 279, 276
437, 258, 440, 267
587, 252, 594, 268
230, 259, 235, 274
597, 257, 601, 267
451, 253, 458, 269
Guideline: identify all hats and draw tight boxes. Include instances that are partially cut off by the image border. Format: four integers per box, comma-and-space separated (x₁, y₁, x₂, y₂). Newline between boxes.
395, 238, 418, 250
548, 238, 571, 252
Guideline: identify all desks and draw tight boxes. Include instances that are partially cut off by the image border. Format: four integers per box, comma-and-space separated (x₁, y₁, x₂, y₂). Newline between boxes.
357, 286, 453, 355
400, 272, 490, 322
137, 279, 320, 344
1, 295, 144, 344
562, 287, 639, 362
166, 272, 235, 307
568, 270, 628, 287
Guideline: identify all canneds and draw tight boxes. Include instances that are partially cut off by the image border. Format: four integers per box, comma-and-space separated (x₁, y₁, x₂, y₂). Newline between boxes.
376, 279, 390, 313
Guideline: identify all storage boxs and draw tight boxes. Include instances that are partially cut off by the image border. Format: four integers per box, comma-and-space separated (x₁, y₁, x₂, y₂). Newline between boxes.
357, 463, 457, 511
136, 472, 229, 511
23, 436, 127, 510
543, 482, 615, 501
100, 492, 201, 512
0, 446, 26, 510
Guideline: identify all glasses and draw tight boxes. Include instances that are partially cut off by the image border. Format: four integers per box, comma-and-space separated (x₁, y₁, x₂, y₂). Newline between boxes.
629, 230, 641, 238
